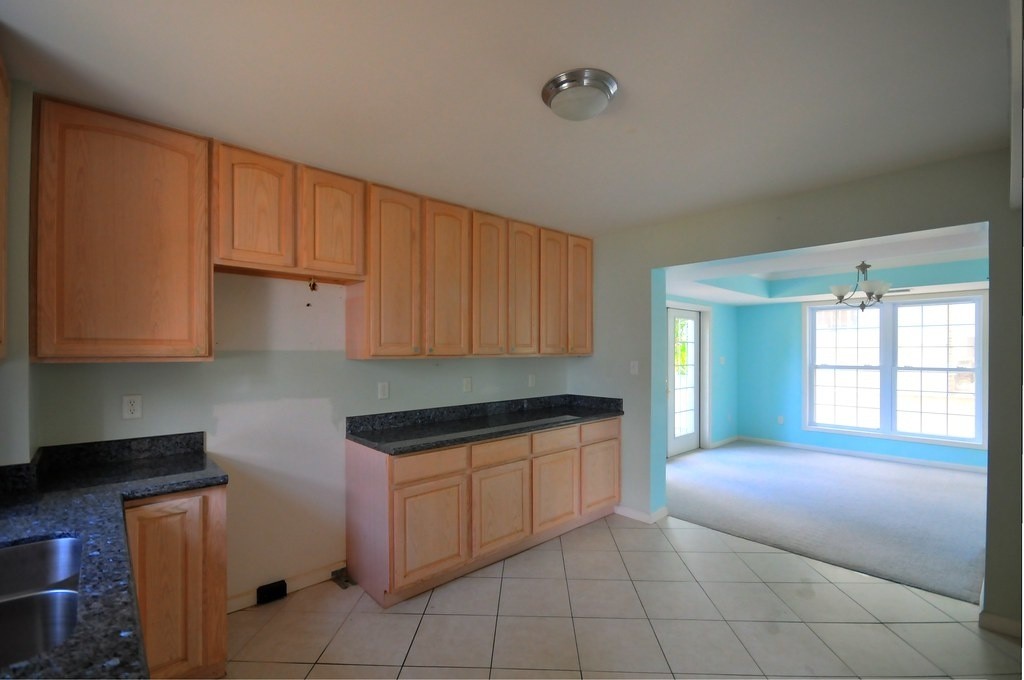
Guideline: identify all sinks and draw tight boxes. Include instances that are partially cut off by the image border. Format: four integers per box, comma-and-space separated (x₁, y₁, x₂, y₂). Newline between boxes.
0, 537, 83, 596
0, 591, 78, 668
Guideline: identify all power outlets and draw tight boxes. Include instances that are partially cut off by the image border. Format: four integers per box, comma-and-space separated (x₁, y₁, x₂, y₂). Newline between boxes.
122, 396, 142, 419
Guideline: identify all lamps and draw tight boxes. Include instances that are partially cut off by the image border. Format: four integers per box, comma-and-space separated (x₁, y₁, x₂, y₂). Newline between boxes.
832, 262, 889, 311
543, 67, 618, 119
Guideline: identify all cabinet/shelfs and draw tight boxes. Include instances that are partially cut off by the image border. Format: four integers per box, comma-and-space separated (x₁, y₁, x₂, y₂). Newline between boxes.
345, 418, 623, 610
30, 99, 595, 366
122, 487, 231, 680
